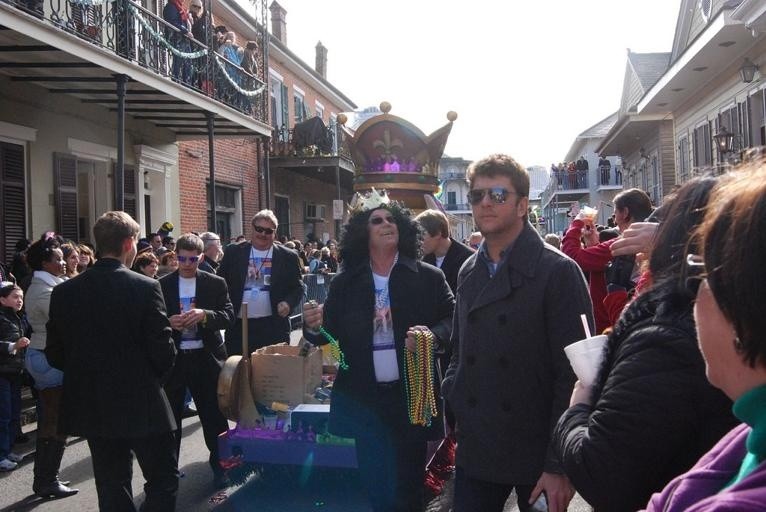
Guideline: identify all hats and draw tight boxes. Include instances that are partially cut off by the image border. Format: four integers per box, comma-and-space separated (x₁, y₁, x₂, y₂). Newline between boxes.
138, 241, 153, 253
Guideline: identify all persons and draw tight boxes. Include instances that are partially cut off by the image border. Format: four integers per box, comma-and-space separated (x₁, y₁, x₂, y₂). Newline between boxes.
0, 232, 96, 497
110, 1, 262, 113
412, 209, 476, 297
470, 232, 483, 251
44, 210, 179, 512
547, 188, 654, 336
448, 158, 593, 510
645, 148, 764, 510
552, 176, 726, 512
279, 235, 339, 274
132, 230, 224, 280
218, 209, 305, 358
156, 234, 231, 477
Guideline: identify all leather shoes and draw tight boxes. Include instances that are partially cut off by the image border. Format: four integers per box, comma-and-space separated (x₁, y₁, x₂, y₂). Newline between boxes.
33, 479, 79, 500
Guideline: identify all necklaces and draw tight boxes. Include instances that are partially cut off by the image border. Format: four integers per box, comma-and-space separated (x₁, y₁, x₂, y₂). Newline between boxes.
316, 323, 349, 372
404, 330, 438, 428
369, 253, 398, 307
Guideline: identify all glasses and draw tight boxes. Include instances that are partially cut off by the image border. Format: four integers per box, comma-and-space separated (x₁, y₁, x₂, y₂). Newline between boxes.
466, 186, 518, 205
254, 224, 276, 234
177, 255, 201, 263
157, 240, 175, 246
369, 216, 398, 225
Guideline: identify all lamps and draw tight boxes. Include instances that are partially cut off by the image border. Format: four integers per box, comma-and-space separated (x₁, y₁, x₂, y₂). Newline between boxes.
711, 124, 737, 154
734, 56, 759, 83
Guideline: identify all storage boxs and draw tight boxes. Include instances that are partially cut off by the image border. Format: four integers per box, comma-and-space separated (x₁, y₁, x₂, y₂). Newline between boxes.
250, 344, 323, 409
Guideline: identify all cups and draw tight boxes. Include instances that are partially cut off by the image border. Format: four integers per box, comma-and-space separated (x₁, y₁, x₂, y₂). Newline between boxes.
584, 205, 598, 220
562, 333, 612, 391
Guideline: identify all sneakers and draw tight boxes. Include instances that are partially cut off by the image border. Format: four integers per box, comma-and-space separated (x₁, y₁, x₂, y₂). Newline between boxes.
1, 453, 25, 471
